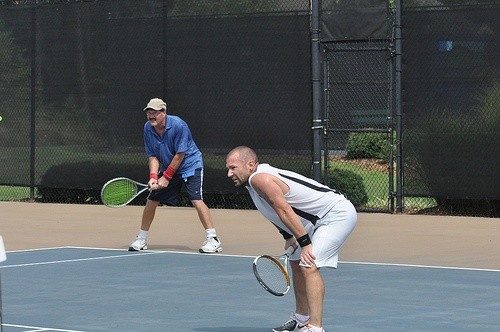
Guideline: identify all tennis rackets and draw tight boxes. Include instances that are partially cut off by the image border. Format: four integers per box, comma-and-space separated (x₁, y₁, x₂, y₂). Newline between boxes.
253, 243, 296, 297
100, 176, 162, 208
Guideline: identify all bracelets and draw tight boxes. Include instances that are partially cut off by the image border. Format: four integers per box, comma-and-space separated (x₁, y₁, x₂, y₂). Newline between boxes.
279, 230, 293, 239
149, 173, 158, 180
296, 233, 312, 248
163, 166, 176, 180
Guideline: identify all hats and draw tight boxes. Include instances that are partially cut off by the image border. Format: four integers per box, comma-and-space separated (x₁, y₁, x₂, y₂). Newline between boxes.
143, 98, 167, 113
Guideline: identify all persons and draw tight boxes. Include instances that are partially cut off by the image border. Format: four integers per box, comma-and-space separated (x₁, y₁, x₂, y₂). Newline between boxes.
226, 146, 357, 332
128, 98, 223, 253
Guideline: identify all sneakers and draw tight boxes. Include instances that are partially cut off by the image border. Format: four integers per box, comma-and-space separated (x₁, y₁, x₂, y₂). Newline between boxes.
128, 239, 148, 251
199, 236, 222, 253
272, 312, 310, 332
